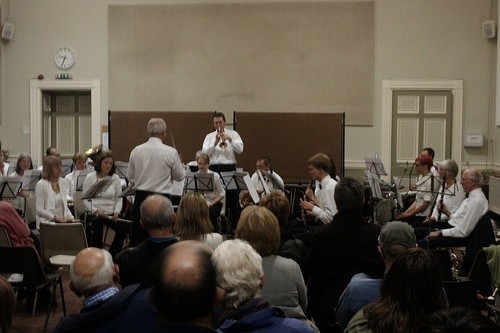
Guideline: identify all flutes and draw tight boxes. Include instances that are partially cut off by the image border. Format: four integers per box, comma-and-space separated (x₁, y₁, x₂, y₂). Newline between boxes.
297, 179, 317, 232
438, 169, 447, 229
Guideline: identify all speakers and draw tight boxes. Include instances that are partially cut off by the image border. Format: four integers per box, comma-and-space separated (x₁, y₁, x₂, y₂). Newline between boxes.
1, 22, 15, 40
482, 21, 496, 39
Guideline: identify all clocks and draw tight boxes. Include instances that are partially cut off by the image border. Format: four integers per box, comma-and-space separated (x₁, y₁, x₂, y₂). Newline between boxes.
54, 47, 76, 69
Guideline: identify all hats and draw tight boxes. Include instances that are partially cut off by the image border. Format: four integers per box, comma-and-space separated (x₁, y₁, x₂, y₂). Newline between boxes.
378, 221, 417, 254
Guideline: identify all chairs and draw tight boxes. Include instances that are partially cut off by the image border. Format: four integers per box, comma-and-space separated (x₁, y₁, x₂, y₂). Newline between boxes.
442, 211, 500, 326
0, 191, 128, 333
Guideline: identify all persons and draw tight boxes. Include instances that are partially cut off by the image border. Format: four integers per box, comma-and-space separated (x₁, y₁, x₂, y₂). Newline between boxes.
334, 220, 500, 333
209, 239, 320, 333
127, 118, 186, 248
47, 147, 64, 157
201, 112, 245, 234
185, 150, 225, 233
287, 152, 344, 247
82, 150, 127, 263
115, 190, 310, 320
250, 157, 285, 199
306, 177, 383, 333
395, 149, 494, 248
52, 247, 163, 333
0, 275, 37, 333
0, 200, 65, 275
65, 151, 98, 212
10, 152, 39, 225
134, 239, 230, 333
35, 155, 81, 230
0, 151, 10, 177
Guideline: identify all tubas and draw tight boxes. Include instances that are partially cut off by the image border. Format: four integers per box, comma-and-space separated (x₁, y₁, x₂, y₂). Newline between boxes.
85, 144, 103, 164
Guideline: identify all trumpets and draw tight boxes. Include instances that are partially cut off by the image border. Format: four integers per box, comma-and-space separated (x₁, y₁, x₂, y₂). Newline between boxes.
217, 126, 228, 148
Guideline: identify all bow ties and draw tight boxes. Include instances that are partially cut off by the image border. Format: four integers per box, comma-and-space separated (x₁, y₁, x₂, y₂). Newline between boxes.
259, 177, 265, 181
465, 192, 469, 198
319, 184, 322, 190
